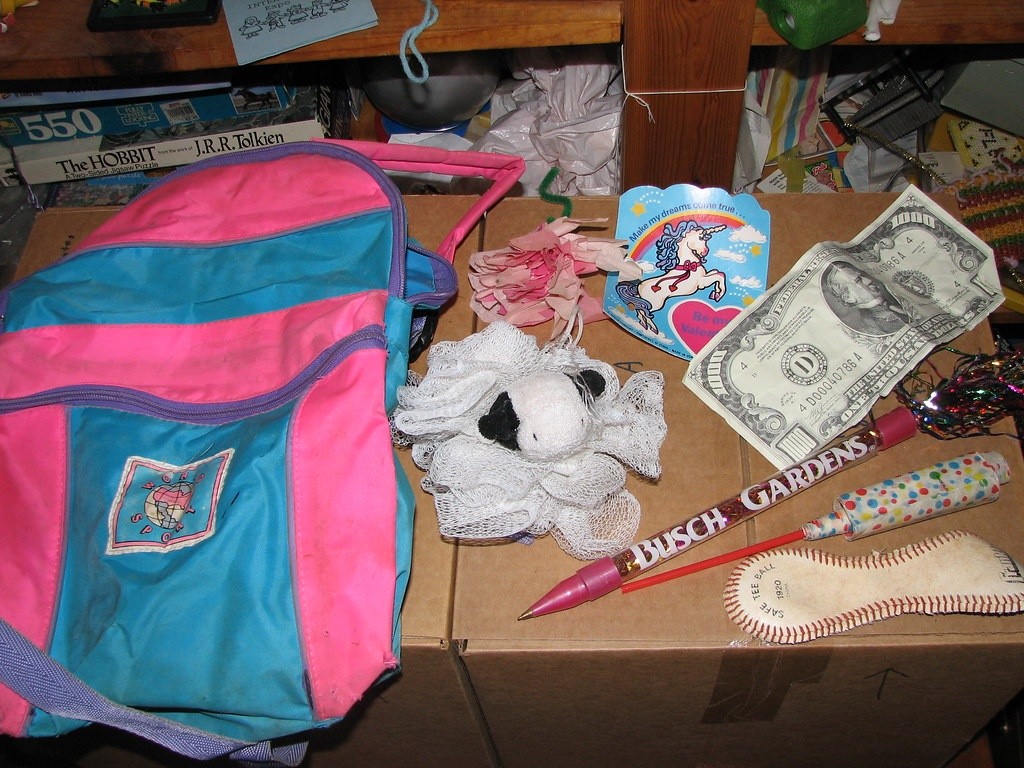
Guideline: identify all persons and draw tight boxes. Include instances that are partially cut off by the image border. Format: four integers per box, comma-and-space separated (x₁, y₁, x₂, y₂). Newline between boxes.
827, 263, 909, 336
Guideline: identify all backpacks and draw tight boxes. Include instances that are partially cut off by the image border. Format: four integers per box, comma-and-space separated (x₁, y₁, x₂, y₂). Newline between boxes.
0, 137, 531, 768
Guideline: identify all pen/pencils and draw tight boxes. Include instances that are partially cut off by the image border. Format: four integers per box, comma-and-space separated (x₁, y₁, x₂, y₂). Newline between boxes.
519, 405, 918, 622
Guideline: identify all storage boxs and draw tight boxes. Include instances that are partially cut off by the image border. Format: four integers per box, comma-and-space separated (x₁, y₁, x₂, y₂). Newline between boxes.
86, 168, 183, 186
0, 67, 336, 185
444, 188, 1024, 768
0, 70, 293, 147
0, 191, 498, 768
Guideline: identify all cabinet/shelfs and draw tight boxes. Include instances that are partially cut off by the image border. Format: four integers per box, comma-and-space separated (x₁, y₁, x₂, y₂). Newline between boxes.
0, 0, 1024, 193
